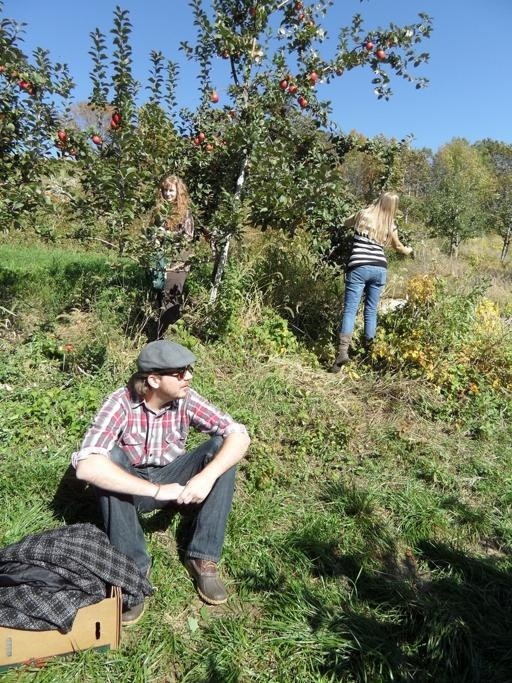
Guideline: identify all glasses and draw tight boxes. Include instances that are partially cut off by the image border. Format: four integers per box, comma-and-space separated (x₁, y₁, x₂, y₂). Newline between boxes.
150, 367, 194, 380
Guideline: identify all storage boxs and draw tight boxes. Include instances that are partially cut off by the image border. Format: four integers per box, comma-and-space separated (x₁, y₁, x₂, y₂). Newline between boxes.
2, 582, 126, 669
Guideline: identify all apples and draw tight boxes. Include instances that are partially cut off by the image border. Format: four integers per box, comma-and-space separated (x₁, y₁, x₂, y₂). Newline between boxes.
193, 93, 236, 152
58, 113, 121, 144
367, 42, 374, 50
378, 51, 385, 58
280, 72, 317, 107
250, 2, 313, 27
0, 65, 33, 94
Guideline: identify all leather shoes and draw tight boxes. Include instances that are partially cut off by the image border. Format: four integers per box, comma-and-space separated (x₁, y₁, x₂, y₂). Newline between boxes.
184, 555, 229, 606
122, 563, 151, 625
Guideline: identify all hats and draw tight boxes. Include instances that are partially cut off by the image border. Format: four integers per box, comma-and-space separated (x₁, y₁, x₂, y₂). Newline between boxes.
136, 340, 195, 371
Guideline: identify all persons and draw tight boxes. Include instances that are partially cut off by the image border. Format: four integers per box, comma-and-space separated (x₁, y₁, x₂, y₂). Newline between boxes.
70, 340, 251, 627
137, 174, 195, 338
327, 189, 414, 373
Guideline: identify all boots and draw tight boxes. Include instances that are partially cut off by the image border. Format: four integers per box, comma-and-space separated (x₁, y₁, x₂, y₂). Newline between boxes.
335, 332, 353, 362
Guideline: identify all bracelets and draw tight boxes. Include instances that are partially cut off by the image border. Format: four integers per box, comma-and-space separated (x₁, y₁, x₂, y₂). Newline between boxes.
152, 482, 160, 499
